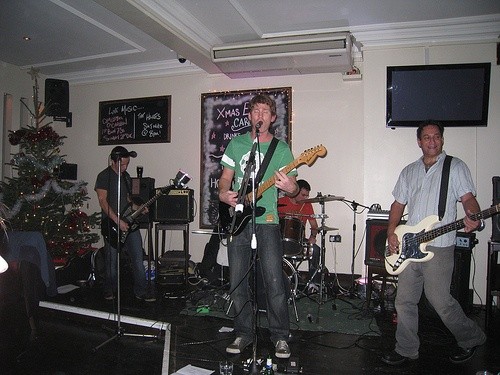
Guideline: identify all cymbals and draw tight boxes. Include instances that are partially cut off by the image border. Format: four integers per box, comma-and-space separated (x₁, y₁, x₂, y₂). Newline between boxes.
310, 226, 339, 230
297, 196, 345, 203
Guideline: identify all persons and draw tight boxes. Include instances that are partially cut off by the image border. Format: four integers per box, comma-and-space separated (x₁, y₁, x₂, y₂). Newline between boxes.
95, 146, 155, 301
381, 120, 487, 365
219, 95, 300, 358
277, 179, 321, 294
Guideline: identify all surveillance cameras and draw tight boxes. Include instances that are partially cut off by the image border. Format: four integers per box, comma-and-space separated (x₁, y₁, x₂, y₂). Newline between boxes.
178, 54, 186, 63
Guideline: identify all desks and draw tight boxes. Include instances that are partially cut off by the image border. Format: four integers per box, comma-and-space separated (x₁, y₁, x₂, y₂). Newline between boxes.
155, 223, 189, 289
365, 265, 398, 314
125, 223, 152, 289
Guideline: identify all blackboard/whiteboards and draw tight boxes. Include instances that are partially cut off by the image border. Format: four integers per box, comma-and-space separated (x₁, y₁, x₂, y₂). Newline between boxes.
97, 94, 172, 146
199, 85, 292, 229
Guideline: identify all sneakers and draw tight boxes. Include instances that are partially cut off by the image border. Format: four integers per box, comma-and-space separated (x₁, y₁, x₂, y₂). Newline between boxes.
275, 340, 291, 358
225, 336, 253, 353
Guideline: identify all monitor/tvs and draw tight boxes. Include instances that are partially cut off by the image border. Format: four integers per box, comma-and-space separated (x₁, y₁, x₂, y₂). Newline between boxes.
386, 62, 491, 126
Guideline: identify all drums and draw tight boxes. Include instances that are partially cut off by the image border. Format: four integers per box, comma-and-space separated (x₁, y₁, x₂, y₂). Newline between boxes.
278, 216, 305, 256
249, 256, 299, 303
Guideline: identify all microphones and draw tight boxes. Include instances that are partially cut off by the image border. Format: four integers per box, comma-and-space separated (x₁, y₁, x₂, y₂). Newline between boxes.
254, 120, 264, 128
112, 151, 137, 158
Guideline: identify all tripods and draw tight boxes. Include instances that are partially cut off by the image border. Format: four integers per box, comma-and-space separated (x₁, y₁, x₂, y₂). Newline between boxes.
293, 200, 370, 324
90, 157, 160, 352
199, 267, 228, 291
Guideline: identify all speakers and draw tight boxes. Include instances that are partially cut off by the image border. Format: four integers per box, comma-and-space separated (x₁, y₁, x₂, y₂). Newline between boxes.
485, 176, 500, 332
153, 189, 194, 224
130, 177, 155, 223
44, 78, 70, 116
415, 233, 476, 326
364, 219, 409, 266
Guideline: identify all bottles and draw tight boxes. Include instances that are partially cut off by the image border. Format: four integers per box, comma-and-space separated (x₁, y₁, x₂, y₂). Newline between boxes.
151, 257, 156, 280
143, 260, 148, 280
264, 354, 275, 375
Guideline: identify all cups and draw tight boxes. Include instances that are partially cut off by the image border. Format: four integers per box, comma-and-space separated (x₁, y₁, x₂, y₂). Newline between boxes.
219, 361, 233, 375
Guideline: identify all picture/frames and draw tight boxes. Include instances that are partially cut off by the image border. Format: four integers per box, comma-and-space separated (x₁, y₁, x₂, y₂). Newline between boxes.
199, 86, 292, 229
98, 95, 173, 146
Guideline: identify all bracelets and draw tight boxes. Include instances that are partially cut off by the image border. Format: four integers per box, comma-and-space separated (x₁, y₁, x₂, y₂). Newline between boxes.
479, 219, 484, 231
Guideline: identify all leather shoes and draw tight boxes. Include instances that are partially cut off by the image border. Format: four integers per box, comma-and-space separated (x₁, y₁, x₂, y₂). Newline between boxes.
449, 345, 481, 363
381, 350, 414, 365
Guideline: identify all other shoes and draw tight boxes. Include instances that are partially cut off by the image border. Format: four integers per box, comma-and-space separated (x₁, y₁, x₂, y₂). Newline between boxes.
136, 295, 156, 301
103, 292, 115, 300
289, 283, 300, 299
308, 287, 318, 297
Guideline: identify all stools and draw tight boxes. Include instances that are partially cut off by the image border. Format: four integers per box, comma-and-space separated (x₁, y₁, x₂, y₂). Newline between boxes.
284, 255, 309, 302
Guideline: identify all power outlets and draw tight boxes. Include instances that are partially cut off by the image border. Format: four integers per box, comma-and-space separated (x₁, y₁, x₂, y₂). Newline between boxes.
329, 235, 341, 243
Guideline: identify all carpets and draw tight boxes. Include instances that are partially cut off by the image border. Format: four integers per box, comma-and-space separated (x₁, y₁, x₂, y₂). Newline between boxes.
180, 287, 379, 337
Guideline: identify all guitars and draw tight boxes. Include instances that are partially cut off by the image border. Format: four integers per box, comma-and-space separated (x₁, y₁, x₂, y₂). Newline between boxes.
219, 145, 327, 236
384, 203, 500, 275
102, 185, 173, 248
